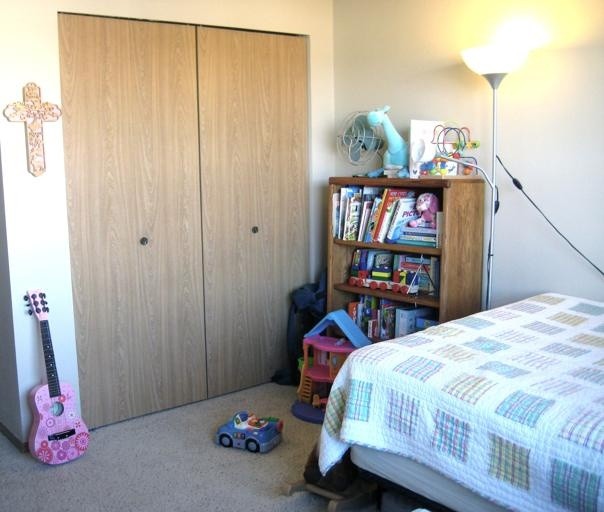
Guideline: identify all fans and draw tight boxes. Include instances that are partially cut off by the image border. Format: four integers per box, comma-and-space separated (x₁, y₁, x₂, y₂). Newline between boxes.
335, 110, 387, 178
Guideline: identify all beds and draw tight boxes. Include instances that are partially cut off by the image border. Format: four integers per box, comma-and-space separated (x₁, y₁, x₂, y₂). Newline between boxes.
316, 292, 604, 512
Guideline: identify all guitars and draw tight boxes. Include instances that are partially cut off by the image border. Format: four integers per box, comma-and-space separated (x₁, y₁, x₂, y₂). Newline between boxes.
24, 289, 90, 465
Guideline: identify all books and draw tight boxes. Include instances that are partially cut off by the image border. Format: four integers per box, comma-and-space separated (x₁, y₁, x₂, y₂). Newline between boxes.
306, 471, 367, 502
331, 185, 444, 342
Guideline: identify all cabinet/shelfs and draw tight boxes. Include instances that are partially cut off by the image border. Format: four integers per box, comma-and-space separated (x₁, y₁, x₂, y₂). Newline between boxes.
59, 12, 312, 432
325, 176, 486, 343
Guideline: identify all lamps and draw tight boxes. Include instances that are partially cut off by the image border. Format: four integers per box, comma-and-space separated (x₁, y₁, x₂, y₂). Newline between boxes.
412, 43, 523, 316
